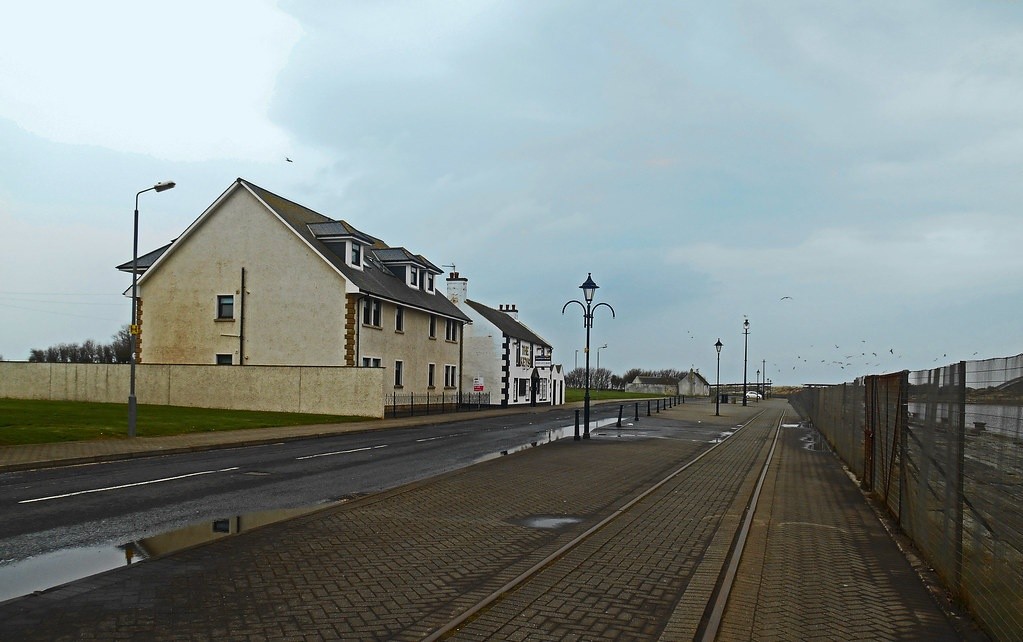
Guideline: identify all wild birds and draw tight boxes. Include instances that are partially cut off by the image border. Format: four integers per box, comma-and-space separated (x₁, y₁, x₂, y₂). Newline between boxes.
286, 157, 293, 162
781, 295, 792, 301
775, 340, 978, 372
688, 330, 693, 339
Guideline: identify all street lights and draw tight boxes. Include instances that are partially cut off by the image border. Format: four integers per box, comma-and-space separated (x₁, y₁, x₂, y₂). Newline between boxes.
756, 369, 760, 403
762, 359, 766, 400
596, 342, 607, 368
742, 319, 751, 406
714, 338, 724, 416
574, 347, 579, 371
128, 180, 176, 439
562, 273, 615, 440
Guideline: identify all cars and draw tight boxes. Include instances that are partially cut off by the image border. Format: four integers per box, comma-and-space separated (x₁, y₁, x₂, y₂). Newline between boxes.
746, 391, 762, 400
